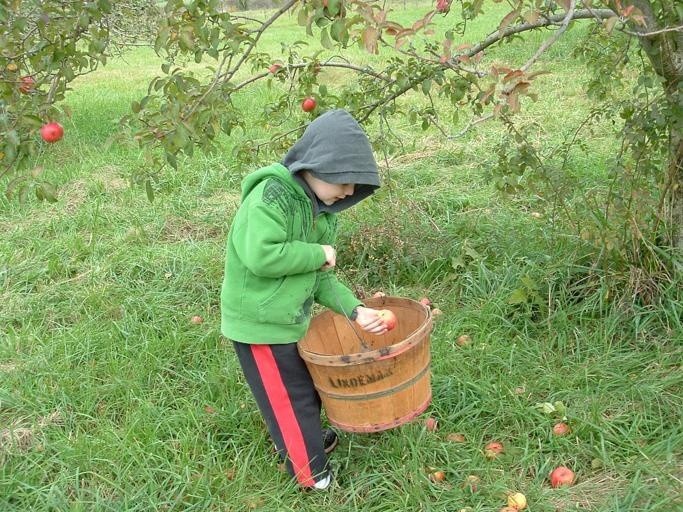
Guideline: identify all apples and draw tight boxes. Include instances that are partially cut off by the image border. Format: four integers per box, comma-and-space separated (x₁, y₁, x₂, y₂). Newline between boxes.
270, 64, 281, 74
426, 418, 576, 512
377, 309, 397, 331
41, 123, 63, 142
302, 98, 316, 112
421, 298, 430, 306
457, 335, 470, 346
19, 77, 33, 95
431, 309, 442, 315
373, 291, 384, 299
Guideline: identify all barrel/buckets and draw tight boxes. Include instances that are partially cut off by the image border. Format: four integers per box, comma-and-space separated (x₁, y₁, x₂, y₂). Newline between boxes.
298, 260, 432, 432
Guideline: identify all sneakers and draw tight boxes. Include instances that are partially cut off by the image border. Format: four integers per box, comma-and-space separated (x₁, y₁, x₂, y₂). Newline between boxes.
269, 427, 341, 469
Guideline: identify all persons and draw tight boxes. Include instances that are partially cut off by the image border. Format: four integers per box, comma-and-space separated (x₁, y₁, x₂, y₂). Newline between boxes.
217, 107, 392, 494
